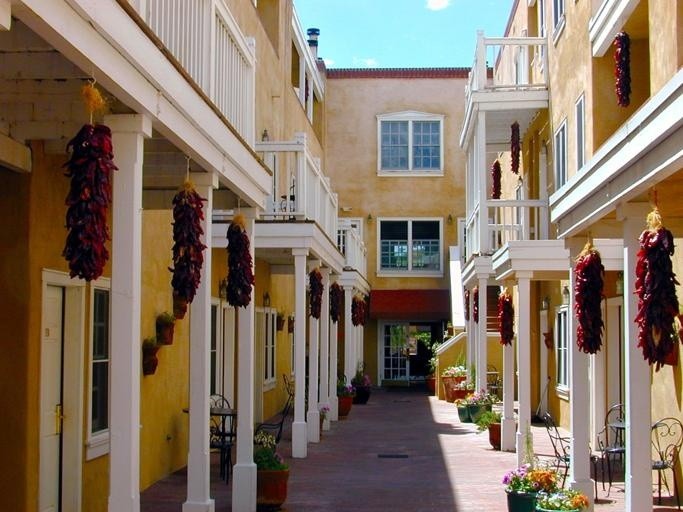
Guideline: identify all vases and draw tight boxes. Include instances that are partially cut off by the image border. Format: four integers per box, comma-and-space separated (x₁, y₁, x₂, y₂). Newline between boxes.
457, 403, 492, 423
256, 470, 288, 512
338, 386, 371, 420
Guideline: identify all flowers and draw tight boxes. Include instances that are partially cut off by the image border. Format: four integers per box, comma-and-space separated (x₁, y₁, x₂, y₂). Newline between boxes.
252, 429, 289, 471
336, 359, 372, 397
499, 419, 590, 512
439, 349, 498, 407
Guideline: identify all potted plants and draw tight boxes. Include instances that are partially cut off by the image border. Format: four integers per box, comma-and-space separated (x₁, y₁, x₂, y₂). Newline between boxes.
474, 410, 518, 451
425, 341, 442, 394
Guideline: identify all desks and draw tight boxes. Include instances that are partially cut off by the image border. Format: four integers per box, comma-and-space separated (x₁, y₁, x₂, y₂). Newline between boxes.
181, 407, 236, 481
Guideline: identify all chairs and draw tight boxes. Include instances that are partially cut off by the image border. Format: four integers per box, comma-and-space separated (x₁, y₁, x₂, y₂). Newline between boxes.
254, 407, 286, 453
281, 372, 294, 414
542, 402, 683, 510
209, 393, 233, 486
